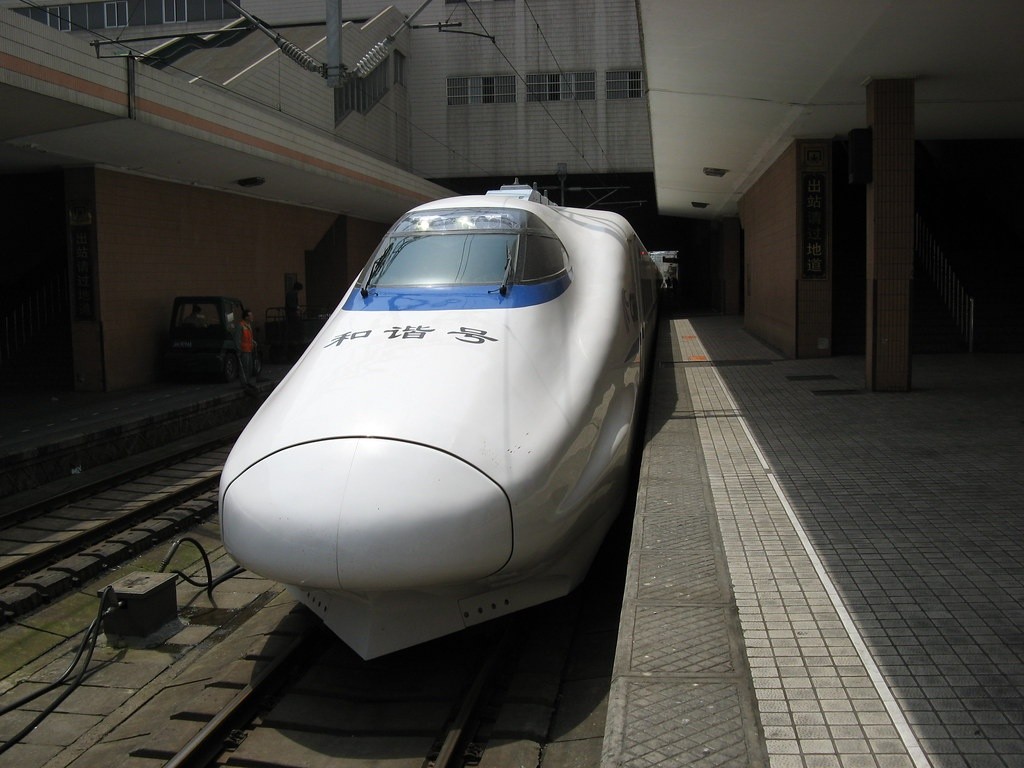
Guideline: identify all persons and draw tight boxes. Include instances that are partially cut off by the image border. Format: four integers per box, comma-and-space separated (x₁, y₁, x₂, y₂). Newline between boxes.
232, 310, 257, 389
184, 305, 210, 328
285, 282, 303, 321
665, 276, 678, 294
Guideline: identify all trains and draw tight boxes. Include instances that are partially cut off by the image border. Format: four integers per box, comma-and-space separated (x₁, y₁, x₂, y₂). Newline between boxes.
213, 177, 665, 666
